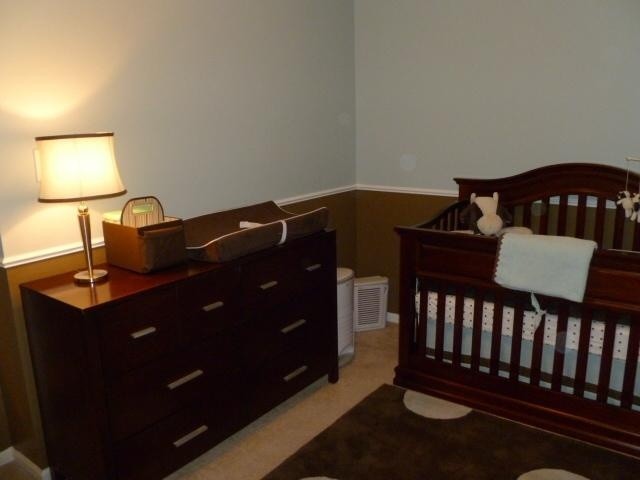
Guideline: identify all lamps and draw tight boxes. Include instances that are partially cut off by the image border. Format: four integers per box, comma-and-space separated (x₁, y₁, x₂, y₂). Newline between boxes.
35, 133, 127, 286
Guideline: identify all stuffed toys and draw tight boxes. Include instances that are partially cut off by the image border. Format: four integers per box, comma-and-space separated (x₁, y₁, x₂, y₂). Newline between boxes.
629, 194, 640, 223
615, 190, 636, 219
470, 192, 502, 236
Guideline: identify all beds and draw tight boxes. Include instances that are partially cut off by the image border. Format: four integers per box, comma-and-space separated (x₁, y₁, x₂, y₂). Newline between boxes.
394, 164, 640, 461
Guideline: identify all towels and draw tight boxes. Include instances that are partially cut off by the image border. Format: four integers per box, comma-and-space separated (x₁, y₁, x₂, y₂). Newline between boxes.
494, 232, 597, 303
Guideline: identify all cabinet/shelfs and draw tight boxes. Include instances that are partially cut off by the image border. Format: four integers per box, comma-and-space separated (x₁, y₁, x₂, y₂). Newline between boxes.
19, 227, 340, 480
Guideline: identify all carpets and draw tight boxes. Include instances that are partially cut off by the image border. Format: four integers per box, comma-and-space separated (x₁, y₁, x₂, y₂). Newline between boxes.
260, 383, 640, 480
